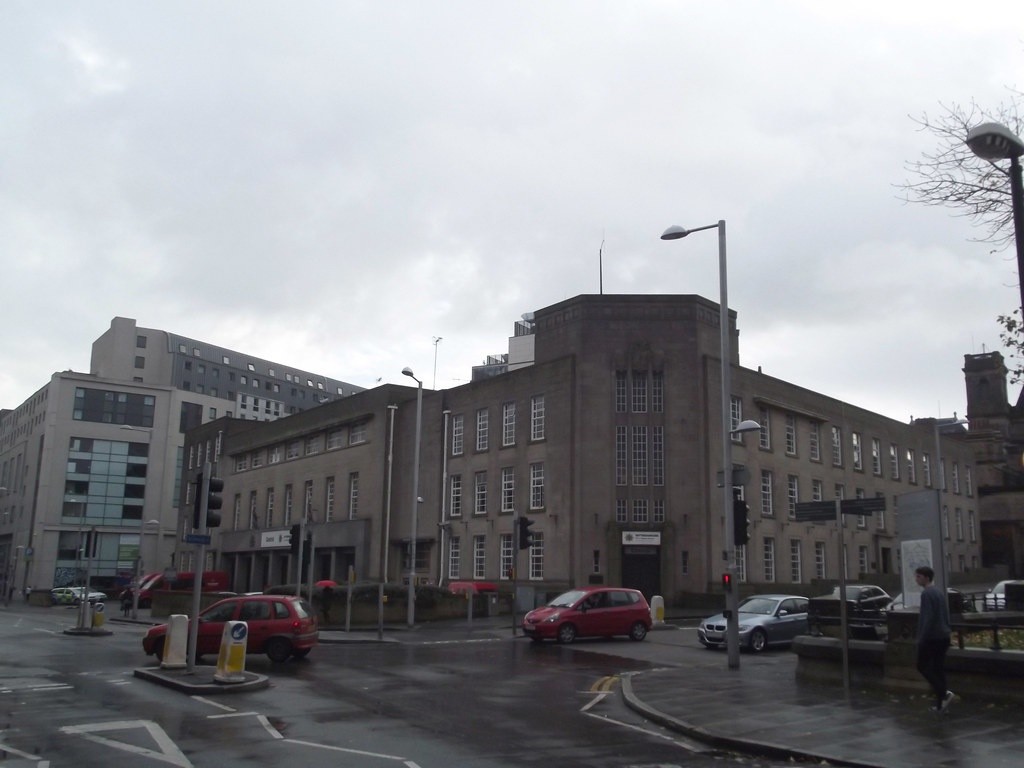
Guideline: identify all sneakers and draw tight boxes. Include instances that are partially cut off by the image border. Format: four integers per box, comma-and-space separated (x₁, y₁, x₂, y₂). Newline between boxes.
936, 689, 955, 714
928, 705, 949, 714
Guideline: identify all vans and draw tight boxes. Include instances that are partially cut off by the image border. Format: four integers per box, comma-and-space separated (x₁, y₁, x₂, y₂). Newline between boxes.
448, 582, 495, 596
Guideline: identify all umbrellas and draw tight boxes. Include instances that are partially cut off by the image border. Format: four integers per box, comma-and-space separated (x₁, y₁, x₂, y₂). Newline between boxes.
316, 580, 336, 588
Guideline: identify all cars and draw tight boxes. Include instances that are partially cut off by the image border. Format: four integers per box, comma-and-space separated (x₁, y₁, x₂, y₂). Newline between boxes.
522, 587, 652, 643
50, 586, 106, 606
881, 588, 974, 612
985, 579, 1024, 610
698, 585, 888, 654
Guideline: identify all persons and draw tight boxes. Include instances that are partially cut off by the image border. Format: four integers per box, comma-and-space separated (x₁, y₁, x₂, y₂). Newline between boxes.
26, 586, 31, 601
123, 587, 132, 617
320, 586, 332, 626
914, 566, 954, 713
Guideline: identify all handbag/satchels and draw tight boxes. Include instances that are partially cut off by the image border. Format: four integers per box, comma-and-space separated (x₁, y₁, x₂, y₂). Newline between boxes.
124, 599, 133, 605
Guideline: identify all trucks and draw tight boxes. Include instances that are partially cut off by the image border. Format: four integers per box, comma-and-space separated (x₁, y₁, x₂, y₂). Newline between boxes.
119, 571, 230, 609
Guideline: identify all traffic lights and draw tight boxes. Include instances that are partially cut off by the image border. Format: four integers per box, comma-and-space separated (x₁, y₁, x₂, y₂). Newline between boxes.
723, 573, 731, 590
289, 524, 300, 555
194, 473, 223, 528
734, 501, 750, 545
520, 516, 533, 549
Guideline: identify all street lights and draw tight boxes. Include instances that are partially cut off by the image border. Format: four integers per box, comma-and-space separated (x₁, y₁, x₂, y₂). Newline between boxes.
120, 424, 160, 619
10, 546, 23, 599
662, 220, 762, 668
70, 498, 84, 587
402, 367, 422, 627
933, 419, 968, 630
965, 120, 1024, 321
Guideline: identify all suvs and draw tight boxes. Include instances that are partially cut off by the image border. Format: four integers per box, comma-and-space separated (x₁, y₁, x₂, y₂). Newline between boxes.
143, 595, 318, 662
